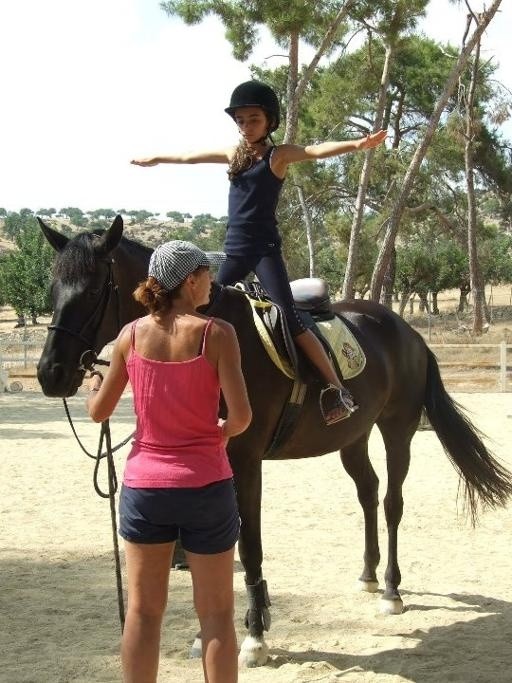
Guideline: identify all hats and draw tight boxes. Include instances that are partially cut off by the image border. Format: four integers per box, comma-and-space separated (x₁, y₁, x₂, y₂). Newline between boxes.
148, 240, 227, 291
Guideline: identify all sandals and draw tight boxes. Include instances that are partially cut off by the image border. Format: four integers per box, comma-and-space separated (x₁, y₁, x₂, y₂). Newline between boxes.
325, 390, 360, 422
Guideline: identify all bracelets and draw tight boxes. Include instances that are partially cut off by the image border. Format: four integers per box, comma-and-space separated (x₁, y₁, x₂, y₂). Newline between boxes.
90, 370, 102, 380
90, 387, 99, 392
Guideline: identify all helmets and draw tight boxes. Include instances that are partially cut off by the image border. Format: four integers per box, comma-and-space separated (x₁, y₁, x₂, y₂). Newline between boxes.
224, 80, 280, 132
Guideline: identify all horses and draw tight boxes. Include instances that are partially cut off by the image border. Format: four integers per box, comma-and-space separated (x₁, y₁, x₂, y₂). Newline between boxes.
31, 211, 512, 668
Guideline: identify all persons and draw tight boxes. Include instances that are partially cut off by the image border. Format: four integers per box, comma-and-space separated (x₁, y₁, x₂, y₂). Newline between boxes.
131, 79, 388, 426
86, 239, 253, 683
